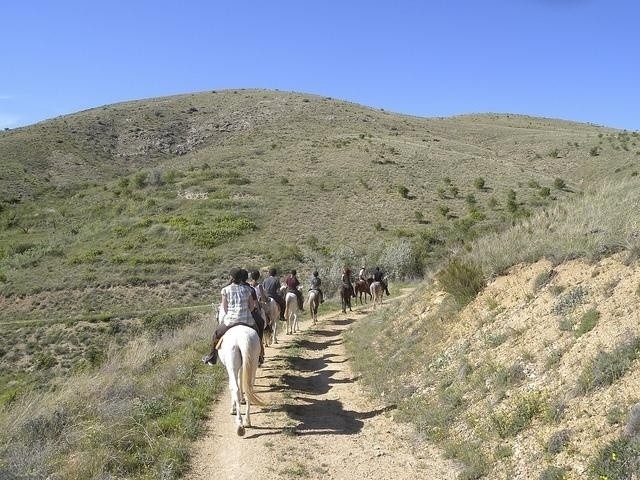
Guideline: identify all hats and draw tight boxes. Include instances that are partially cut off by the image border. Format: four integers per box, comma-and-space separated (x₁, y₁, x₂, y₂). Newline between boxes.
268, 269, 277, 277
313, 271, 318, 277
251, 270, 260, 280
290, 270, 296, 275
229, 268, 248, 282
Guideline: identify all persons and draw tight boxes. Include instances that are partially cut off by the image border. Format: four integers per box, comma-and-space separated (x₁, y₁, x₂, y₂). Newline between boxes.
341, 266, 357, 300
286, 270, 304, 312
308, 270, 325, 304
359, 265, 369, 289
261, 267, 287, 322
248, 270, 271, 312
241, 269, 265, 358
201, 267, 265, 367
372, 266, 391, 296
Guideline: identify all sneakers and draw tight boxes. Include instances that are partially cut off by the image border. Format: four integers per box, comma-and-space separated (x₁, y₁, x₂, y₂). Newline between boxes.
201, 357, 217, 367
320, 300, 325, 304
279, 317, 288, 322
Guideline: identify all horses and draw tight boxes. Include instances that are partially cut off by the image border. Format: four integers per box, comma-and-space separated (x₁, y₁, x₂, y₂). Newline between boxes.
282, 284, 305, 335
368, 276, 389, 310
256, 282, 288, 368
354, 276, 374, 307
337, 282, 354, 315
307, 289, 320, 325
210, 301, 263, 436
